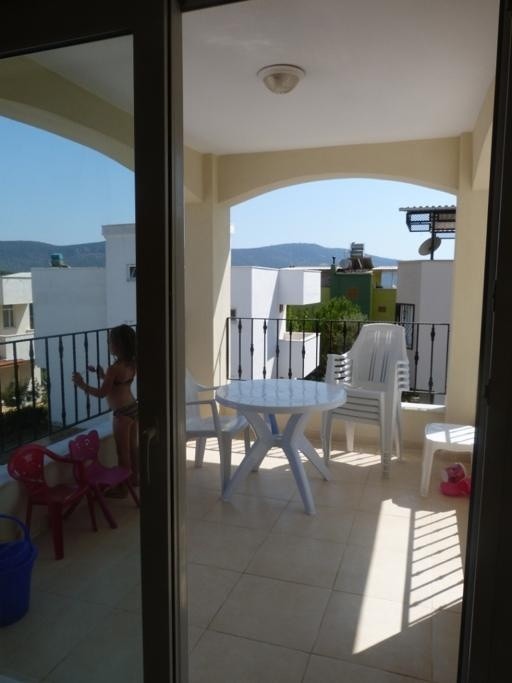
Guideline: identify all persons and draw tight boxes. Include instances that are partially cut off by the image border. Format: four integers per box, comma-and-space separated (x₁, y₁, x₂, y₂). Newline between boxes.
71, 324, 140, 493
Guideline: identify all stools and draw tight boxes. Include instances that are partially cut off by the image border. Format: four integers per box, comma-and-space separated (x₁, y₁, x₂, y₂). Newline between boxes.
415, 418, 475, 500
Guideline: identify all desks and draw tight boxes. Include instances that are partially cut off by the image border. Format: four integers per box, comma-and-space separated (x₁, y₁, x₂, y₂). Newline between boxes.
214, 378, 352, 521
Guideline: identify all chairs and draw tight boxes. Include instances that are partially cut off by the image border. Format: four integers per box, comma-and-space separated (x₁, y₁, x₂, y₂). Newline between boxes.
319, 322, 412, 484
67, 426, 141, 534
180, 361, 261, 497
7, 440, 101, 561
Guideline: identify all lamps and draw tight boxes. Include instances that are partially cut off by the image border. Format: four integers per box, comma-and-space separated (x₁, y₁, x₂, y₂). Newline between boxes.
255, 63, 308, 95
